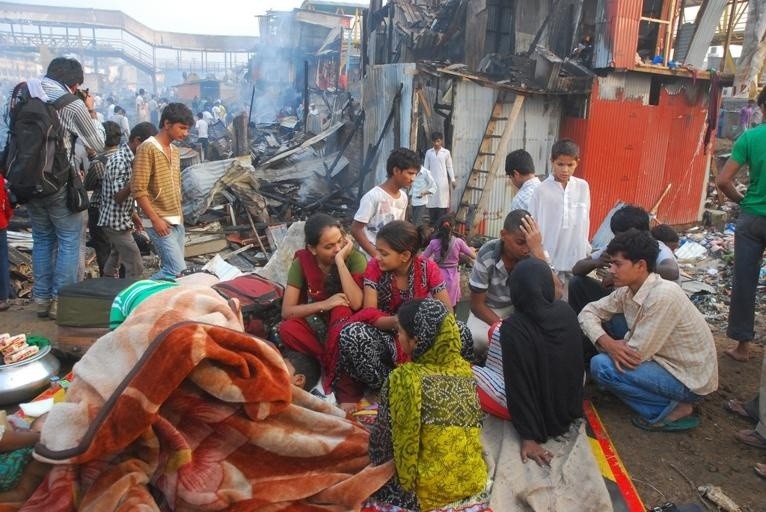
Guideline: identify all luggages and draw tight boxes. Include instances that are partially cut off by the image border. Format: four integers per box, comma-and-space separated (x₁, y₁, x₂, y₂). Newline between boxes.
216, 272, 288, 337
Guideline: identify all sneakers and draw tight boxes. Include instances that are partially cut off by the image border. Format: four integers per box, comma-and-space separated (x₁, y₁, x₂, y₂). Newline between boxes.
36, 301, 48, 317
48, 298, 59, 320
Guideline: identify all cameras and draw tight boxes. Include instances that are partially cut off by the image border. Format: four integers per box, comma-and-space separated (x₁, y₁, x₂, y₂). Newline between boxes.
74, 88, 89, 102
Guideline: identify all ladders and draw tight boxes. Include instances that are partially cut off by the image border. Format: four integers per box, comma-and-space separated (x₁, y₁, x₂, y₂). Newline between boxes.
454, 89, 525, 237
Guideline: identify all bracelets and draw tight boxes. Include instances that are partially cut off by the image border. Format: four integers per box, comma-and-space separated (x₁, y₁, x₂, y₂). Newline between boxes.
320, 300, 324, 313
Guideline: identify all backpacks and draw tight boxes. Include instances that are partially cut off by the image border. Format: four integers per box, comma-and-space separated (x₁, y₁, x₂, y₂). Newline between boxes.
5, 84, 81, 203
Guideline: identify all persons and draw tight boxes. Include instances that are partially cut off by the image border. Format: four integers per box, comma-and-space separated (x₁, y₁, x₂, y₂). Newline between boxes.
368, 297, 495, 510
278, 212, 367, 397
471, 87, 766, 468
83, 103, 195, 282
0, 407, 43, 494
336, 216, 475, 402
407, 149, 437, 226
0, 57, 250, 159
420, 216, 478, 319
2, 58, 106, 319
424, 131, 456, 233
347, 147, 422, 262
1, 176, 11, 312
282, 350, 320, 392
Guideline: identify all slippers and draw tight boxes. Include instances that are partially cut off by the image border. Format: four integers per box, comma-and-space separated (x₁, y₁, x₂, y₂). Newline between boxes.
724, 398, 756, 421
735, 427, 765, 449
631, 412, 700, 431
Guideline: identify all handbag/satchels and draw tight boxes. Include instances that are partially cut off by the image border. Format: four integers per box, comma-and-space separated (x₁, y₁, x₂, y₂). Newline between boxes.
67, 171, 91, 213
